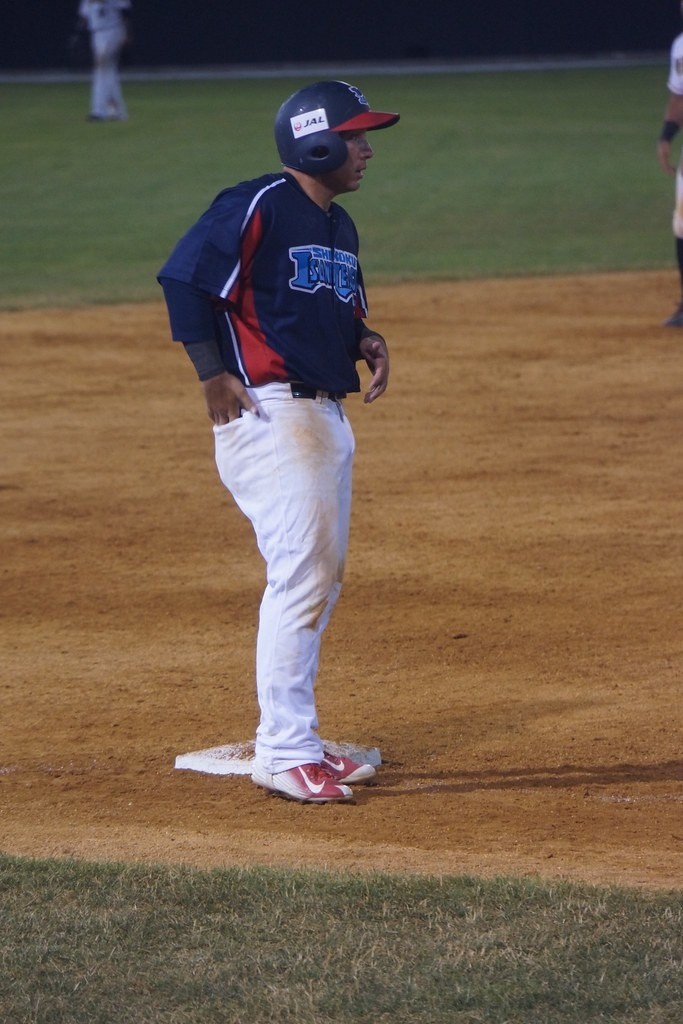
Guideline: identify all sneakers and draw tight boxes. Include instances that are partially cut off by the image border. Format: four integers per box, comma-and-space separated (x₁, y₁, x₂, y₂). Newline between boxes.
250, 763, 354, 801
321, 749, 377, 782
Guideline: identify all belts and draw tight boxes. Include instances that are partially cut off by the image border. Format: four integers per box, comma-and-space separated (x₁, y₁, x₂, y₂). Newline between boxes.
291, 384, 347, 399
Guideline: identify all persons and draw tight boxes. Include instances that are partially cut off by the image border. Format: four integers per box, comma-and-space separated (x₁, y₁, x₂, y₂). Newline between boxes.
659, 31, 683, 329
79, 1, 133, 123
156, 81, 402, 804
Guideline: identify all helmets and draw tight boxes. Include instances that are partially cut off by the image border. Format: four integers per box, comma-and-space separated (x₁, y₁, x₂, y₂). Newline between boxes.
274, 81, 401, 173
